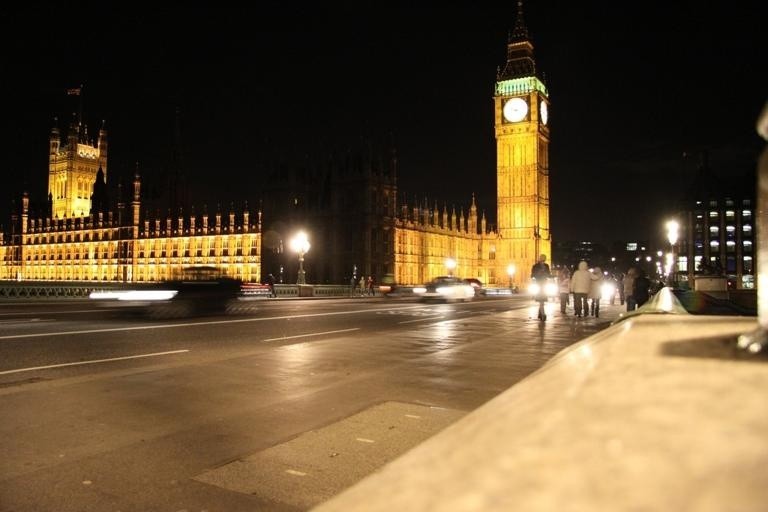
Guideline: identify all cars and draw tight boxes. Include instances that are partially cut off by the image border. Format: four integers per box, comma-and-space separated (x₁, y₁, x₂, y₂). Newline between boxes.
413, 274, 477, 303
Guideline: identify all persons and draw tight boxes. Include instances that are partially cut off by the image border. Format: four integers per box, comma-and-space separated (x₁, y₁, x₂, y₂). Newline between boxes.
367, 275, 376, 297
556, 259, 668, 318
358, 275, 366, 297
528, 254, 552, 323
262, 273, 277, 298
350, 274, 357, 298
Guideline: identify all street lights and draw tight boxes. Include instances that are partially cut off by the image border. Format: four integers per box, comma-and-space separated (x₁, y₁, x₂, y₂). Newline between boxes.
287, 228, 312, 287
665, 219, 679, 272
